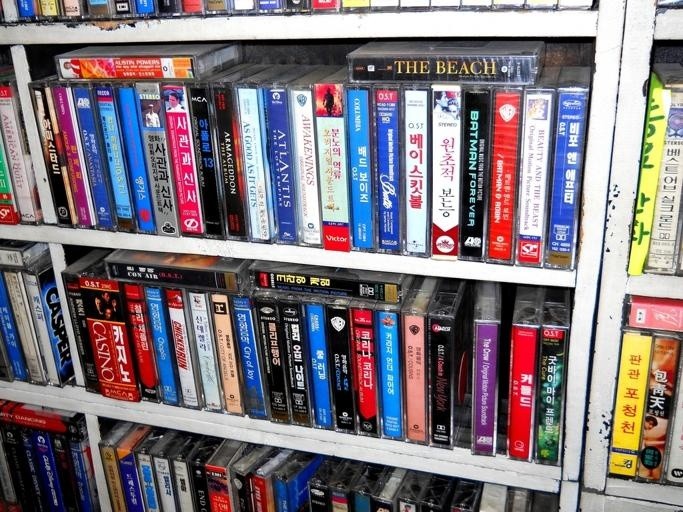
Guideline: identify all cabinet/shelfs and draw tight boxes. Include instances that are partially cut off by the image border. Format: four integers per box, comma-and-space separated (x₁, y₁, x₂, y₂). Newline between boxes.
582, 1, 683, 501
2, 2, 625, 512
578, 488, 681, 511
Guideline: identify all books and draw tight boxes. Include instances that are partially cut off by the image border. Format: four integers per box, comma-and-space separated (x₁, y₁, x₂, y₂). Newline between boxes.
1, 399, 560, 512
628, 64, 682, 275
1, 1, 597, 24
346, 41, 547, 87
1, 243, 572, 467
53, 44, 244, 79
0, 67, 590, 269
608, 297, 682, 484
